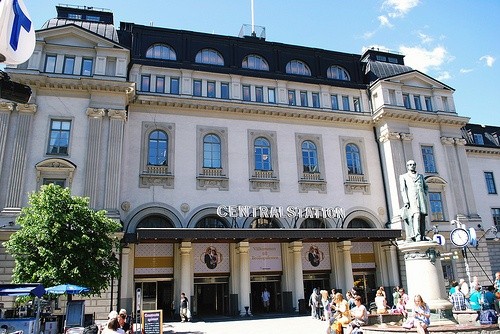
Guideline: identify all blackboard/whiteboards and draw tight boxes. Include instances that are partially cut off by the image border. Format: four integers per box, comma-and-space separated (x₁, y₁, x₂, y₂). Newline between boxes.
141, 309, 163, 334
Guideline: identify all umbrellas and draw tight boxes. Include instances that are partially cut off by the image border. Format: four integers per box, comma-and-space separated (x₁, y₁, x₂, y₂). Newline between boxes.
44, 283, 91, 324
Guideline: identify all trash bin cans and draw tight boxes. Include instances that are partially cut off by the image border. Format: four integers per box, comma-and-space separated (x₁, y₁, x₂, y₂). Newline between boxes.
298, 299, 307, 314
44, 315, 59, 334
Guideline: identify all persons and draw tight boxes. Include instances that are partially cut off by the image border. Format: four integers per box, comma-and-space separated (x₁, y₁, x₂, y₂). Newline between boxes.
373, 285, 388, 316
447, 272, 500, 326
448, 286, 468, 312
100, 307, 135, 334
326, 292, 351, 334
308, 288, 358, 323
261, 288, 270, 314
347, 295, 369, 334
399, 159, 434, 245
391, 285, 411, 319
410, 294, 432, 334
179, 291, 191, 323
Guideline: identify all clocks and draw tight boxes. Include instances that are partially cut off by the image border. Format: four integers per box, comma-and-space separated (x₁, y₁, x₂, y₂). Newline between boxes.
451, 228, 469, 247
